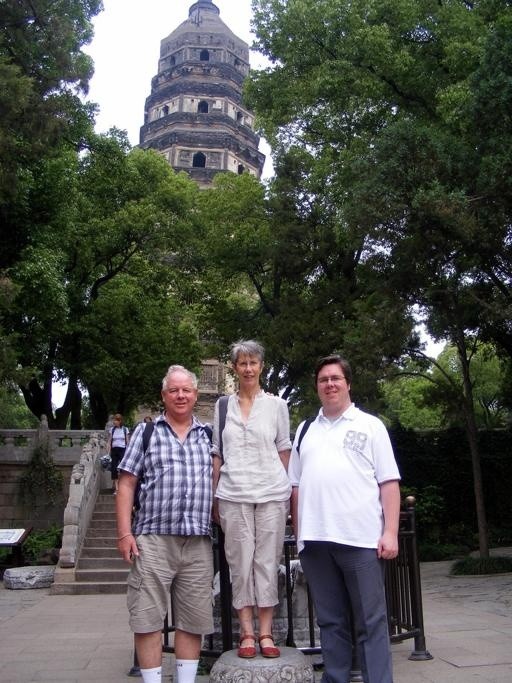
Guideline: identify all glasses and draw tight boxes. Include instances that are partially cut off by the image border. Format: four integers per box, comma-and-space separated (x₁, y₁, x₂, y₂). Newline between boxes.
316, 375, 345, 386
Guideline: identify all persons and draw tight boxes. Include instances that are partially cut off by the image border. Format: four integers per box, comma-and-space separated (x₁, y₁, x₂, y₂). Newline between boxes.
286, 354, 403, 682
105, 414, 131, 493
143, 416, 153, 423
114, 363, 216, 683
207, 338, 294, 657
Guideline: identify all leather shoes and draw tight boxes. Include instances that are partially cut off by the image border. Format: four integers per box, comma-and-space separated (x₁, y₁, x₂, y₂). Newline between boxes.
259, 633, 280, 658
238, 635, 257, 659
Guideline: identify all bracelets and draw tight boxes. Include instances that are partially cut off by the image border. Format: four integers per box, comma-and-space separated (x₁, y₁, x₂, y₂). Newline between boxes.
118, 532, 131, 541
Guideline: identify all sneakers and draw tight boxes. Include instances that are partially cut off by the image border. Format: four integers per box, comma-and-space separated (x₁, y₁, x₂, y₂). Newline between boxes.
113, 489, 118, 495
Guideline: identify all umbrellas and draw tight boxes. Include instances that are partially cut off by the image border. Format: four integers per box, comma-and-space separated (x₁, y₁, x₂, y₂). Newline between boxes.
101, 449, 112, 468
